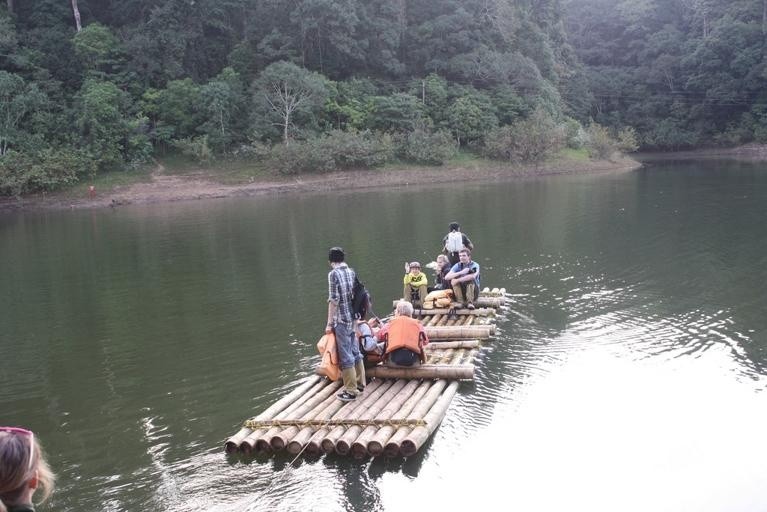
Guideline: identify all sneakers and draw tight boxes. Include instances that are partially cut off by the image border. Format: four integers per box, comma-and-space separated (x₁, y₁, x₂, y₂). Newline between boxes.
454, 302, 464, 309
466, 302, 475, 310
336, 393, 357, 402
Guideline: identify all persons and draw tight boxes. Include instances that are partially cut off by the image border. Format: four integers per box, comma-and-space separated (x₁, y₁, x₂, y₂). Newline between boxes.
441, 222, 473, 266
427, 254, 452, 294
0, 426, 58, 511
443, 248, 481, 309
325, 244, 365, 400
403, 260, 429, 306
378, 301, 428, 369
358, 317, 384, 368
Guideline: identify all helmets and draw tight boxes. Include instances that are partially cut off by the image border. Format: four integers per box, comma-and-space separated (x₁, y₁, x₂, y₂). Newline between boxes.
409, 262, 421, 269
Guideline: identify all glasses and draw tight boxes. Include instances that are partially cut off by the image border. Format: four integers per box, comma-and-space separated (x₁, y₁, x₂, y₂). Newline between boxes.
0, 425, 35, 470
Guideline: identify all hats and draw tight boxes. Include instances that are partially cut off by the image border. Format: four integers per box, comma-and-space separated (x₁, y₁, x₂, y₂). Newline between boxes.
328, 247, 344, 262
448, 222, 459, 231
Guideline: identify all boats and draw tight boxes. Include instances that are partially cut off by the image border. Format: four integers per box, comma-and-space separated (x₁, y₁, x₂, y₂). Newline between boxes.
224, 287, 506, 459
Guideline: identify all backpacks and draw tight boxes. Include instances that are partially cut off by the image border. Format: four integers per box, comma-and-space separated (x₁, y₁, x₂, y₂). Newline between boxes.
349, 275, 371, 321
446, 232, 462, 253
